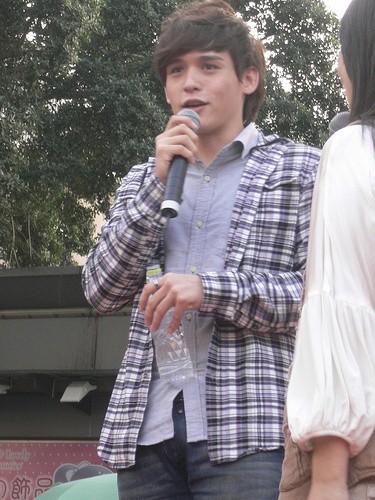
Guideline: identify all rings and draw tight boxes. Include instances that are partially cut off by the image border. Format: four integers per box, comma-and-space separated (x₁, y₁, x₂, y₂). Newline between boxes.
152, 278, 160, 289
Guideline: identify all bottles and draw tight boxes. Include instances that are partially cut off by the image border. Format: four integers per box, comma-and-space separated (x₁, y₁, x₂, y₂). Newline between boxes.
143, 259, 197, 387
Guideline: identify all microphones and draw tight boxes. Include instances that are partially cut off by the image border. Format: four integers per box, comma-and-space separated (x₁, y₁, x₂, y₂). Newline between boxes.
160, 108, 201, 218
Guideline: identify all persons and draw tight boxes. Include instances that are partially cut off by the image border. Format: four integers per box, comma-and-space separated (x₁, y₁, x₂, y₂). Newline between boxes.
278, 0, 375, 500
81, 0, 321, 500
